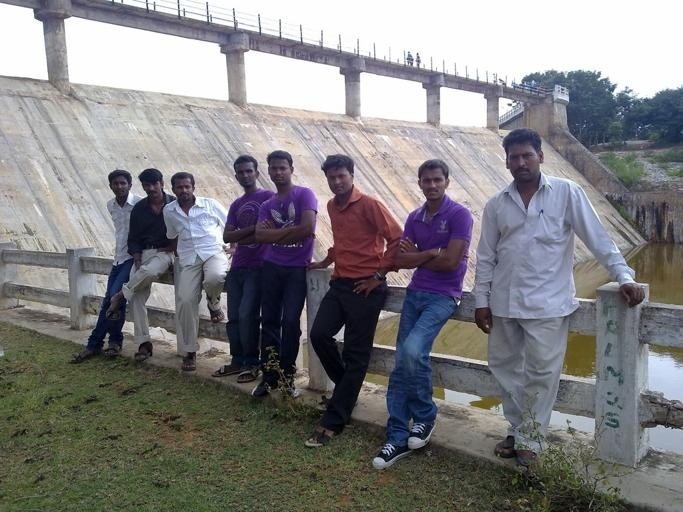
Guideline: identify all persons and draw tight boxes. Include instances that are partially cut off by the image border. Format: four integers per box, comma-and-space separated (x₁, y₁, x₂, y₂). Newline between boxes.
252, 150, 317, 398
163, 174, 234, 372
473, 128, 646, 469
71, 169, 144, 362
106, 169, 175, 362
305, 156, 404, 447
211, 155, 276, 382
372, 160, 472, 468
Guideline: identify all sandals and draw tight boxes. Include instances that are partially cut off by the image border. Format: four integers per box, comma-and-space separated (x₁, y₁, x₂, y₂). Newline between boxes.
494, 436, 515, 458
135, 341, 152, 362
103, 341, 121, 355
68, 346, 101, 364
211, 364, 295, 399
207, 303, 225, 323
182, 352, 196, 372
106, 293, 122, 321
304, 426, 337, 447
516, 450, 537, 467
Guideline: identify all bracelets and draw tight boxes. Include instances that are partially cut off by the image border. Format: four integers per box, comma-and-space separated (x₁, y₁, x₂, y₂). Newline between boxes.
437, 246, 441, 256
376, 272, 385, 281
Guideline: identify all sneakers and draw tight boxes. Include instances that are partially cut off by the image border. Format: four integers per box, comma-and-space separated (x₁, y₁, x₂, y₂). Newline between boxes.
408, 419, 438, 449
372, 443, 414, 469
